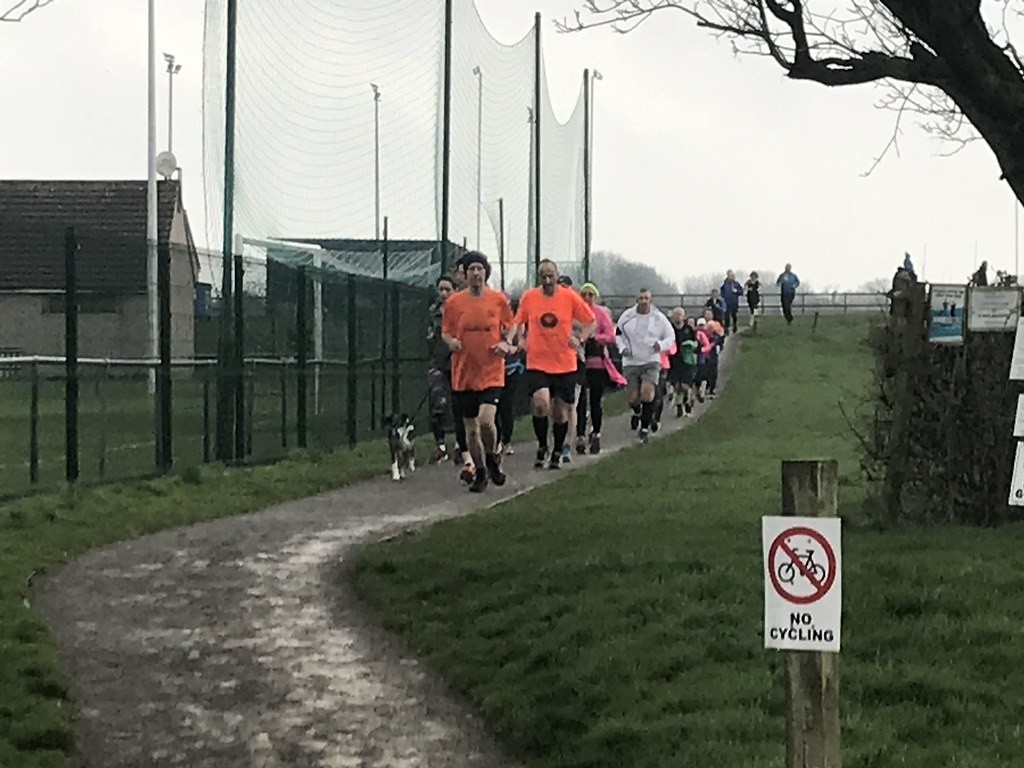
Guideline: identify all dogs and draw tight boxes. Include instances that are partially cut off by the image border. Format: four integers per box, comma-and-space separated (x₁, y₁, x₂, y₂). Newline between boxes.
388, 413, 415, 480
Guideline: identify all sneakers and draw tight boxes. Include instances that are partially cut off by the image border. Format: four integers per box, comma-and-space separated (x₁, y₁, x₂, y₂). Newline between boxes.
429, 445, 448, 465
454, 442, 462, 465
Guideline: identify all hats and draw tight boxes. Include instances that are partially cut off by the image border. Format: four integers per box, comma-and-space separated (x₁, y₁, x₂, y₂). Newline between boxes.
557, 276, 572, 285
697, 318, 706, 326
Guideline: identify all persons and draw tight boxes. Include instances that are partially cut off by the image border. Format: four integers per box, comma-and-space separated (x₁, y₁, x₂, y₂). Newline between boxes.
776, 264, 799, 326
744, 271, 762, 326
706, 289, 723, 325
424, 250, 726, 493
720, 269, 742, 335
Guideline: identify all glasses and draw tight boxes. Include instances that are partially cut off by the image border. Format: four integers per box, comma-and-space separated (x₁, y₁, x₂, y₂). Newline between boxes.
582, 293, 594, 298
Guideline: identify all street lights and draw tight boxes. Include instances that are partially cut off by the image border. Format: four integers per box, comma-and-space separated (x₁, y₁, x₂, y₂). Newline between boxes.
584, 70, 603, 276
371, 83, 385, 250
164, 47, 182, 162
525, 108, 534, 277
472, 64, 481, 260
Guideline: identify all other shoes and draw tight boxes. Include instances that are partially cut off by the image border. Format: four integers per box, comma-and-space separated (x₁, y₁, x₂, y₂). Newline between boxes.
486, 466, 506, 486
630, 413, 661, 440
460, 463, 473, 485
562, 448, 571, 462
590, 433, 601, 454
469, 476, 488, 493
534, 446, 549, 470
733, 327, 737, 333
496, 440, 514, 454
666, 392, 716, 420
724, 330, 729, 335
547, 451, 561, 469
576, 435, 586, 454
